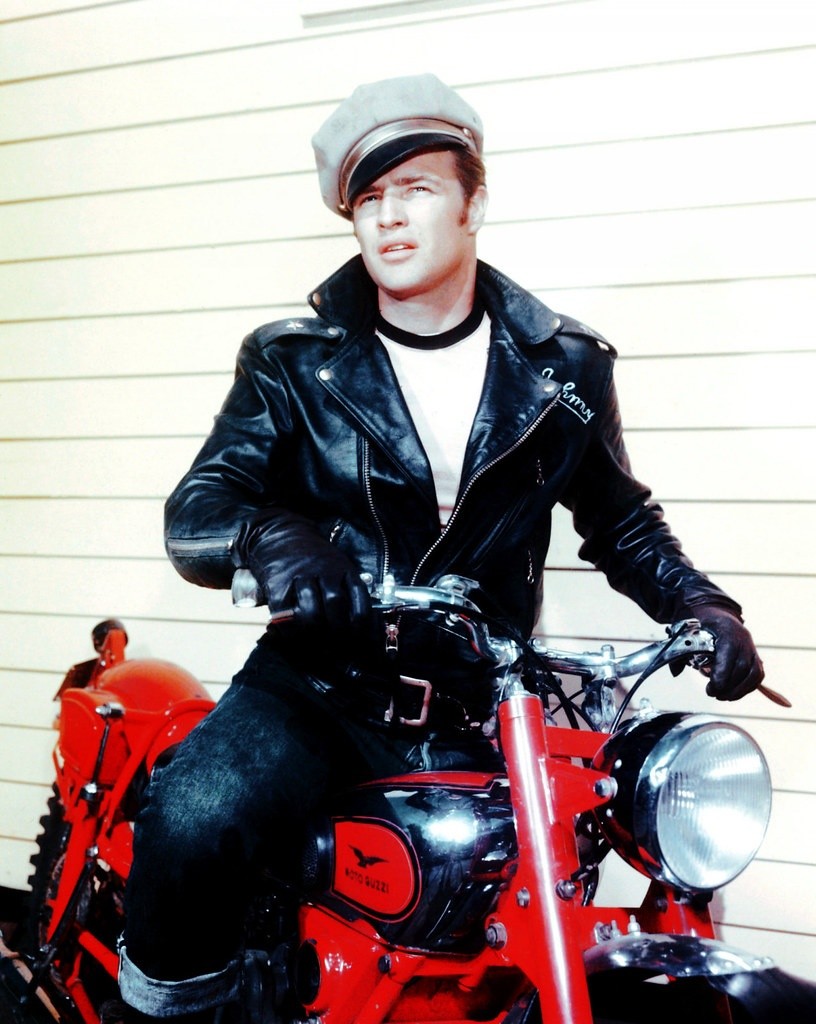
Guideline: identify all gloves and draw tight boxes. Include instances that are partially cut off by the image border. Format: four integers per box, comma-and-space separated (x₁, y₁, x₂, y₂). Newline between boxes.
668, 604, 764, 703
241, 512, 369, 635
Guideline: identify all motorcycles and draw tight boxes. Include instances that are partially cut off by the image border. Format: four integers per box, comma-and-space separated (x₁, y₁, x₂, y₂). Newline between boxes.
0, 570, 816, 1023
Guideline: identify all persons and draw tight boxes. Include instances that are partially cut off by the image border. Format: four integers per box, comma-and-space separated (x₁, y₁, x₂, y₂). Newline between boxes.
102, 73, 765, 1024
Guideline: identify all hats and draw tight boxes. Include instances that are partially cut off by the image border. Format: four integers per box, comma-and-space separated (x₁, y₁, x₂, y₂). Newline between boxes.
311, 72, 482, 219
